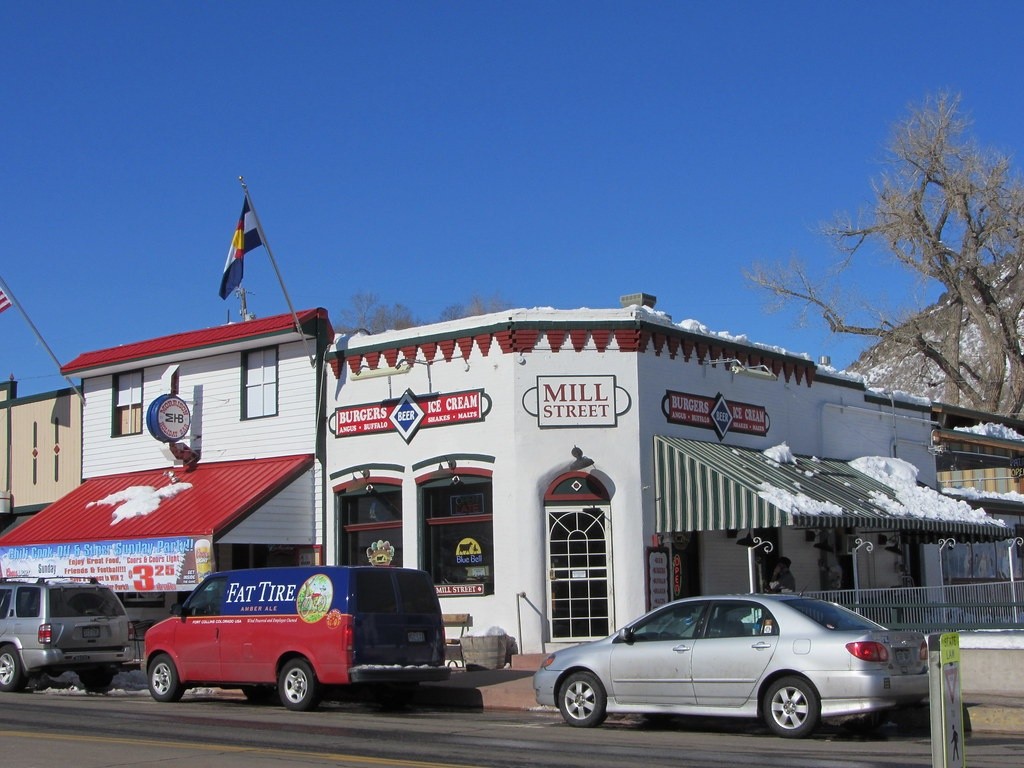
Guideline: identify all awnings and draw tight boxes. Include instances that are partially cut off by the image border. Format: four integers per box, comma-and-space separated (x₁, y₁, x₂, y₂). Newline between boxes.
654, 435, 1013, 543
0, 457, 316, 592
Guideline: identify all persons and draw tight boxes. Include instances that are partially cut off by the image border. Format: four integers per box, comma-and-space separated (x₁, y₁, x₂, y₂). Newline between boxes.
771, 556, 794, 592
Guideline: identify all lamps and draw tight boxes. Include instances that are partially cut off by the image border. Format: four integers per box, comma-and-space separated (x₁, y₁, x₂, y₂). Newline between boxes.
703, 359, 777, 381
351, 359, 431, 383
726, 528, 757, 547
570, 445, 595, 471
806, 531, 833, 553
346, 467, 370, 493
430, 456, 456, 476
879, 534, 902, 555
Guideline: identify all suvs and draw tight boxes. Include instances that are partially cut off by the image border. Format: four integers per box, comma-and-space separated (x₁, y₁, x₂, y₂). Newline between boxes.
0, 574, 133, 692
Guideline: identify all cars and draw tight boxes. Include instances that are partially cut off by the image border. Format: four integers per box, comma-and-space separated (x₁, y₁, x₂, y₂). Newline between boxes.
533, 593, 929, 738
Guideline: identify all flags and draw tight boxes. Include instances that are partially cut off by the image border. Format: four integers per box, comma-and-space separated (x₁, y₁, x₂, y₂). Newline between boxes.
0, 289, 11, 313
219, 195, 261, 300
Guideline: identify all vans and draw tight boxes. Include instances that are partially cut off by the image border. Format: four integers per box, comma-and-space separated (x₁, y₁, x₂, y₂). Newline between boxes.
144, 565, 451, 712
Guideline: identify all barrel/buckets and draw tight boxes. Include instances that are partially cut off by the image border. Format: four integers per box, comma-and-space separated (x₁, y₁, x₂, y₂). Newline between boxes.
460, 635, 507, 671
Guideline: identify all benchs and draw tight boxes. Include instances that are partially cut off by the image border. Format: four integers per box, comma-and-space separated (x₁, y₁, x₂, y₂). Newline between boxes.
443, 614, 470, 668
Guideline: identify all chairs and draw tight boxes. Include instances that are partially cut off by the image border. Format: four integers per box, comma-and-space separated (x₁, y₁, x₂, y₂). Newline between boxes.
132, 619, 157, 658
711, 619, 744, 637
